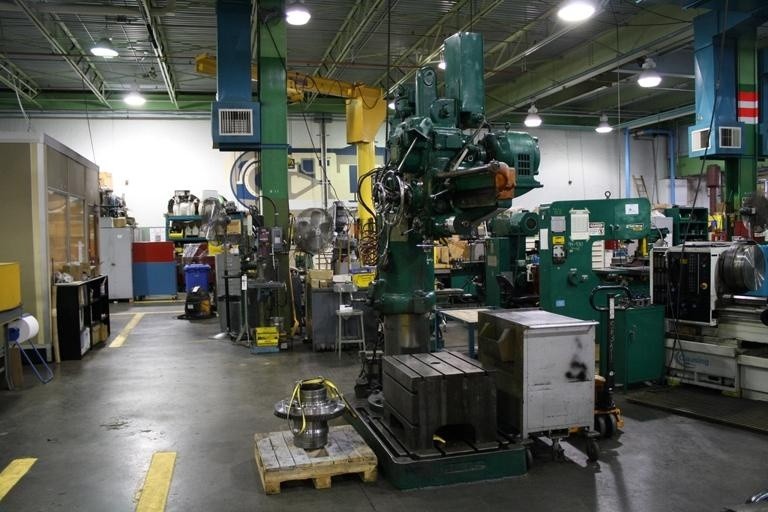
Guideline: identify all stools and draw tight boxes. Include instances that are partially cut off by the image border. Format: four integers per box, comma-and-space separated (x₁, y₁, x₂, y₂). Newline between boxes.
336, 310, 366, 360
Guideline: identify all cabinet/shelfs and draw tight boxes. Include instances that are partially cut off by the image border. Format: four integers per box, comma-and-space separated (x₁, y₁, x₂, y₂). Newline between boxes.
55, 274, 111, 360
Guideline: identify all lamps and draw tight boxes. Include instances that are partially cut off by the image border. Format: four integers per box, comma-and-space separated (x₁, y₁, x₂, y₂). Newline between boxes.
525, 99, 542, 127
123, 80, 146, 108
284, 1, 312, 25
638, 56, 662, 87
595, 111, 614, 134
89, 19, 119, 58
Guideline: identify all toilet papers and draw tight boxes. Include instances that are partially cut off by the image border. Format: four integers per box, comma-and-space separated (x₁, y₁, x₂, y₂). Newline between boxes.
9, 315, 39, 344
